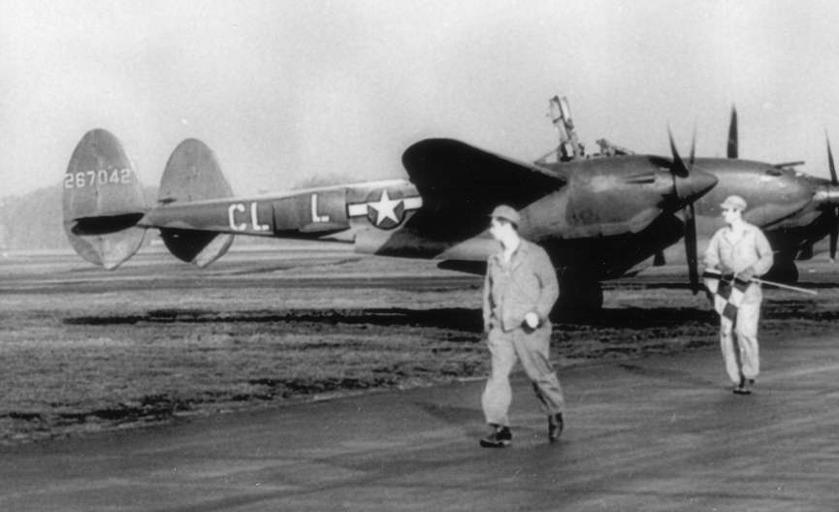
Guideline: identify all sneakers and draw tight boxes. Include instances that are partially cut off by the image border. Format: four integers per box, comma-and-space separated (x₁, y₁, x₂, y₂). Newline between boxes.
547, 412, 563, 440
733, 377, 754, 393
479, 425, 512, 448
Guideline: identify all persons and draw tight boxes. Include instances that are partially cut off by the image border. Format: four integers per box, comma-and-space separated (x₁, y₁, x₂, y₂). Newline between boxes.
479, 205, 564, 446
703, 194, 775, 395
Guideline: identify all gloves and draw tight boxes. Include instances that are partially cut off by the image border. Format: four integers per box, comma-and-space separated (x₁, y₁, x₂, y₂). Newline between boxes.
718, 264, 733, 280
736, 266, 755, 286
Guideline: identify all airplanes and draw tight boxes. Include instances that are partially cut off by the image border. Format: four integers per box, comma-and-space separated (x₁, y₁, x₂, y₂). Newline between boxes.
61, 98, 839, 310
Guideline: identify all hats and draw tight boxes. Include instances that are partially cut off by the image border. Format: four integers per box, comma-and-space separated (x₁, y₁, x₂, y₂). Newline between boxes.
719, 196, 746, 210
488, 204, 522, 228
